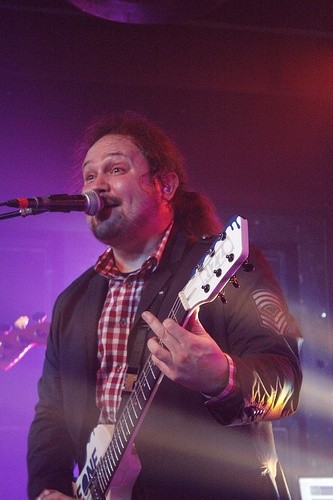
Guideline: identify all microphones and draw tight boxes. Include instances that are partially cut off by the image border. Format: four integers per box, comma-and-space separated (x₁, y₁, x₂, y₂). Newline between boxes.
6, 189, 105, 217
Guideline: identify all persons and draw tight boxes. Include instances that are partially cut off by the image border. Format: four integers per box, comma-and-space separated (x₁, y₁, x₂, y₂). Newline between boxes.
27, 112, 302, 500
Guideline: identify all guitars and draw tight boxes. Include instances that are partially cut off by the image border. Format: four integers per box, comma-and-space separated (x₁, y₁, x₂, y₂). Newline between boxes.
72, 215, 254, 500
